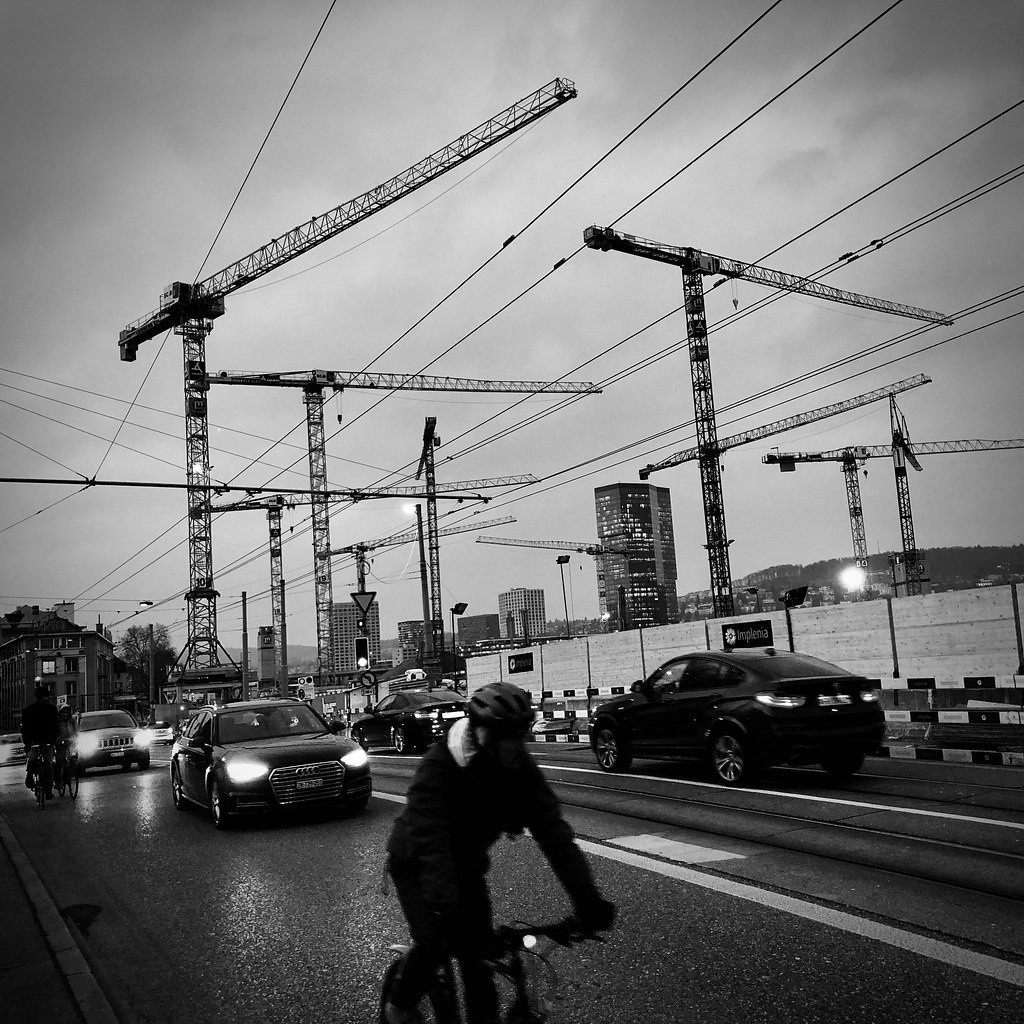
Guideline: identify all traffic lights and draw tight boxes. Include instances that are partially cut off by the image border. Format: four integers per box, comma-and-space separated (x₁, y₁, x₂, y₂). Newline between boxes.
354, 637, 370, 671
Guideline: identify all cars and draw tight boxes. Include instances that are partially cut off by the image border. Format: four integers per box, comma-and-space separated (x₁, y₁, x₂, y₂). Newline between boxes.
174, 719, 190, 742
588, 648, 888, 789
69, 708, 151, 778
145, 721, 176, 746
171, 697, 374, 830
0, 733, 28, 767
350, 685, 472, 756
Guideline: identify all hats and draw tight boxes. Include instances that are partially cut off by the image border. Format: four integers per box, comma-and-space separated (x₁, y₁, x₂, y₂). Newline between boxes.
57, 703, 69, 711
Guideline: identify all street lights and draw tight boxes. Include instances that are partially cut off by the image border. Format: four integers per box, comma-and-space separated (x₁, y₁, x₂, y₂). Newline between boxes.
449, 602, 468, 694
555, 555, 571, 635
778, 586, 808, 653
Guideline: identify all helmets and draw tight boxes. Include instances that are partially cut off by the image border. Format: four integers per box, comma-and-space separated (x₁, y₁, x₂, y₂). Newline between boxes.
462, 683, 535, 724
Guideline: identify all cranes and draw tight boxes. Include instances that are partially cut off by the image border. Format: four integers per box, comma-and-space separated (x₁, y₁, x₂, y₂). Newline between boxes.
118, 74, 581, 667
331, 515, 518, 634
580, 223, 955, 622
204, 367, 605, 686
639, 372, 934, 621
211, 473, 541, 697
474, 535, 632, 635
761, 437, 1023, 603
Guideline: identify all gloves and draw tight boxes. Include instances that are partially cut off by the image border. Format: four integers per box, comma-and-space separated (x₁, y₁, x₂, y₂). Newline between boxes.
573, 895, 616, 932
459, 926, 507, 960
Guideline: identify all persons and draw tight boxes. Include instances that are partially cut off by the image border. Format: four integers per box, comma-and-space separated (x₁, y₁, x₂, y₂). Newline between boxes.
381, 682, 617, 1024
22, 686, 78, 800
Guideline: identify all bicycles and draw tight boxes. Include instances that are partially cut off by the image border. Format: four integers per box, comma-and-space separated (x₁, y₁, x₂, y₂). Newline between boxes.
379, 906, 616, 1024
20, 724, 81, 811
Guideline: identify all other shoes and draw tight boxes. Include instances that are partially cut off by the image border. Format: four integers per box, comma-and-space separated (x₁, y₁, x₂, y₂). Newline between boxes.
26, 778, 35, 788
46, 792, 55, 799
